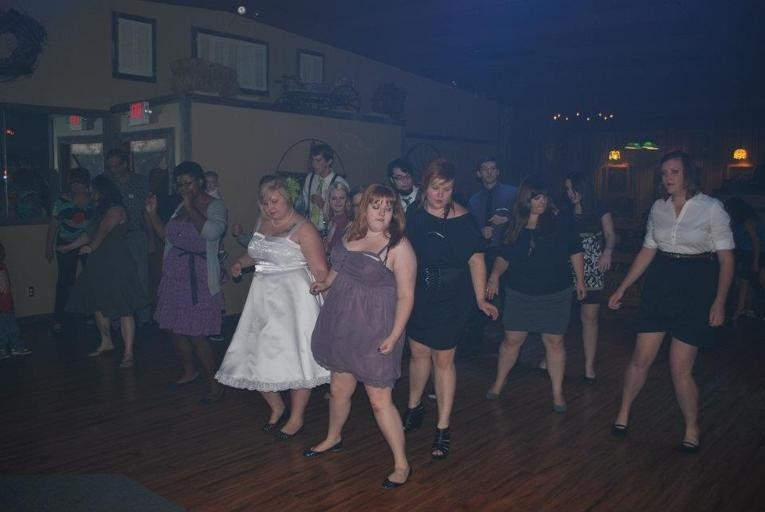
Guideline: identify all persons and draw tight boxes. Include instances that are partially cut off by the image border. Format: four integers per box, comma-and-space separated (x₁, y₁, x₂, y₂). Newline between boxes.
45, 168, 99, 342
232, 194, 306, 247
535, 170, 617, 385
402, 159, 499, 459
212, 176, 331, 440
485, 174, 588, 415
606, 151, 736, 454
0, 244, 33, 360
145, 161, 230, 385
303, 184, 417, 488
101, 149, 156, 330
302, 144, 420, 256
58, 177, 152, 369
455, 156, 518, 358
726, 197, 764, 317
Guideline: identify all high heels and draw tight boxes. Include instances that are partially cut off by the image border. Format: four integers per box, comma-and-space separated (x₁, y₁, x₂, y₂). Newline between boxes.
402, 402, 424, 432
432, 425, 449, 460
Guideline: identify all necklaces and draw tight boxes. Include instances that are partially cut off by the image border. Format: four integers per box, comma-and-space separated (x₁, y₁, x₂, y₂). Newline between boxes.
270, 207, 295, 227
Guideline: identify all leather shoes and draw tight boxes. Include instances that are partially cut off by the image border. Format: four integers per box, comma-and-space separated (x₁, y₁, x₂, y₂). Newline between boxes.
485, 383, 500, 400
680, 441, 698, 451
87, 345, 114, 358
303, 441, 343, 457
172, 373, 199, 386
553, 401, 567, 413
382, 465, 410, 489
612, 422, 627, 436
203, 388, 224, 404
277, 425, 303, 440
586, 376, 597, 385
263, 409, 290, 432
119, 358, 135, 369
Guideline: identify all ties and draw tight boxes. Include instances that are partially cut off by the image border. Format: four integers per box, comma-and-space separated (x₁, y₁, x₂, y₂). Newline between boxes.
310, 180, 324, 225
402, 197, 411, 207
485, 191, 492, 226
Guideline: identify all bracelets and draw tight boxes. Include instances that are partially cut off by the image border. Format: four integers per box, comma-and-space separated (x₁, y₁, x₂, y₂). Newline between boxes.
236, 260, 242, 266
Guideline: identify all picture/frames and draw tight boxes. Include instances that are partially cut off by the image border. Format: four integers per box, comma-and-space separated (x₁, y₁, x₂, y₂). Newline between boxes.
110, 10, 158, 83
295, 47, 325, 89
190, 24, 270, 97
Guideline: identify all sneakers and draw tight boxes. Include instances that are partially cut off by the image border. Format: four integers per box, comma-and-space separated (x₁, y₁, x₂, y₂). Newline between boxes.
1, 349, 10, 359
10, 346, 31, 356
